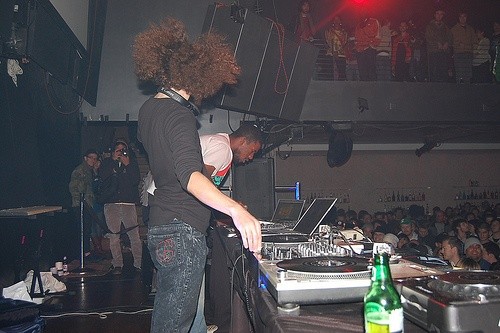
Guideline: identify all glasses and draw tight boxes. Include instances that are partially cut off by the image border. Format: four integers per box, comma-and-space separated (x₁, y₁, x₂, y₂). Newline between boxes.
383, 242, 392, 246
87, 156, 96, 161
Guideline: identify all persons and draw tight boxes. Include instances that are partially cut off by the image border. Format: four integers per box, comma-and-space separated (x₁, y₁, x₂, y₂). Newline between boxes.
292, 0, 315, 46
69, 149, 98, 260
354, 5, 500, 84
86, 147, 119, 257
138, 169, 153, 230
137, 19, 262, 333
324, 15, 349, 81
100, 139, 142, 276
147, 124, 264, 332
318, 201, 500, 272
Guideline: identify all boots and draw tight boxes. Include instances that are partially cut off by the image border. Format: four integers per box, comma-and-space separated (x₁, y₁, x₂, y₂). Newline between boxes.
90, 232, 103, 256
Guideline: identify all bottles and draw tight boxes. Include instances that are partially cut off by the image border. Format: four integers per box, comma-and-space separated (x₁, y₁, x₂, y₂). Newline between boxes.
63, 257, 68, 273
364, 253, 404, 333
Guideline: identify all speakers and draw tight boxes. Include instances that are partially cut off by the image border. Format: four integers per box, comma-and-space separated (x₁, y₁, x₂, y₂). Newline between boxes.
327, 131, 354, 168
254, 125, 293, 158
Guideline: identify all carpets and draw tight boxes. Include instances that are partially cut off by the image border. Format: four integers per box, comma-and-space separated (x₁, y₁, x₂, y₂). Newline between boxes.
65, 259, 112, 276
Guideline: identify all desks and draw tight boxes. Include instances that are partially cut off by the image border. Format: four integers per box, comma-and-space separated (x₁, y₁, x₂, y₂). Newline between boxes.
218, 223, 427, 333
0, 209, 68, 301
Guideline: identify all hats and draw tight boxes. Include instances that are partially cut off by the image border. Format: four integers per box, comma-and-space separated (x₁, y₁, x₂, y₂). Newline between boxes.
115, 137, 128, 149
83, 149, 100, 157
434, 232, 449, 242
374, 226, 385, 233
399, 218, 413, 226
383, 233, 399, 248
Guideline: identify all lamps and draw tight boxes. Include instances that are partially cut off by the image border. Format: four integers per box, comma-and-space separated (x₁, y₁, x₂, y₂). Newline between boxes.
358, 97, 369, 112
416, 141, 438, 156
231, 3, 245, 23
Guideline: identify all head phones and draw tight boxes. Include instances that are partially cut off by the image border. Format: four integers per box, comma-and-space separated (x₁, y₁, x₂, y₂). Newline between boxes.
157, 86, 200, 117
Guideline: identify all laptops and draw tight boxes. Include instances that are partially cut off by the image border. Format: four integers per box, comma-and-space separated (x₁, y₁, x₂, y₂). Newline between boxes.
260, 198, 338, 236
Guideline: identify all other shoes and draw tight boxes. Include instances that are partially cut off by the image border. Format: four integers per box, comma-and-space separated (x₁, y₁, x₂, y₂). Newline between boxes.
205, 324, 218, 333
114, 266, 122, 274
132, 266, 143, 272
143, 292, 155, 305
84, 251, 90, 256
